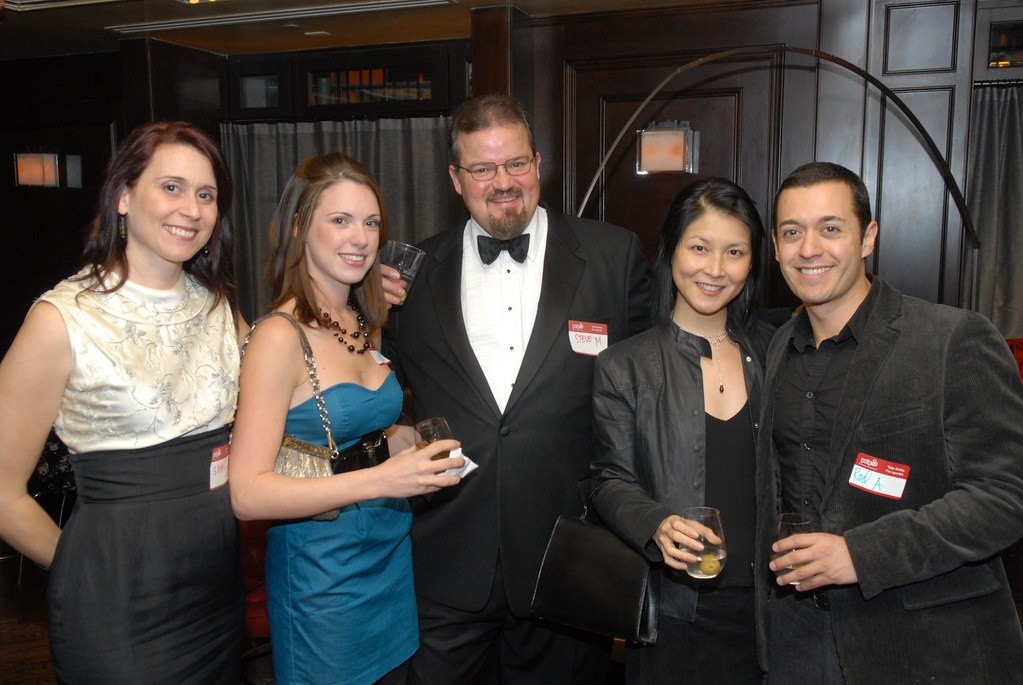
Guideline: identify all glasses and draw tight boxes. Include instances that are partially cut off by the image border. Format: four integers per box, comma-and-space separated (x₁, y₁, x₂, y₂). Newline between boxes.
455, 156, 534, 181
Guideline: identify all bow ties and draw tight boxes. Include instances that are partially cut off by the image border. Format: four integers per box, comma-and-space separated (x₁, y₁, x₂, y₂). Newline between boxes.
478, 233, 530, 265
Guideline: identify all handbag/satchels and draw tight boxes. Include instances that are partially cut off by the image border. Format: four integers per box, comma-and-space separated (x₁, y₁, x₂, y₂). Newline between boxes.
529, 479, 660, 644
228, 312, 341, 521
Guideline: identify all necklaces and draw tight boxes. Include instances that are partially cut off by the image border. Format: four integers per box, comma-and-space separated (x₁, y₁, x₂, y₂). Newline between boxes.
679, 329, 728, 393
319, 300, 370, 355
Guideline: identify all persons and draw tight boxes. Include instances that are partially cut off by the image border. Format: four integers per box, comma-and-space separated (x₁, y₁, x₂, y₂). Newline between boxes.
380, 95, 639, 685
227, 155, 465, 685
594, 177, 776, 685
755, 162, 1023, 684
0, 122, 252, 684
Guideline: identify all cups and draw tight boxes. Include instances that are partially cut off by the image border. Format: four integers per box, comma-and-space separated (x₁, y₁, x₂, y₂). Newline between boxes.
679, 505, 727, 579
772, 510, 821, 584
414, 416, 452, 474
381, 240, 427, 306
